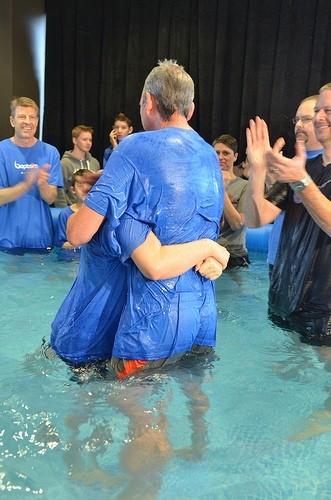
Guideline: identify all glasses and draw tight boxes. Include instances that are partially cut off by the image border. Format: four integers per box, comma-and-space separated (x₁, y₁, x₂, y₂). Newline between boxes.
292, 116, 314, 125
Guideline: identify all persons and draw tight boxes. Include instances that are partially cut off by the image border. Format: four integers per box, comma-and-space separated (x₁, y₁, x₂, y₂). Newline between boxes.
66, 59, 223, 500
0, 97, 134, 262
49, 169, 229, 487
212, 82, 331, 441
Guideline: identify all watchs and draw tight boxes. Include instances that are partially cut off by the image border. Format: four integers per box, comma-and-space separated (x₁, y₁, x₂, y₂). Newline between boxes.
289, 176, 313, 192
